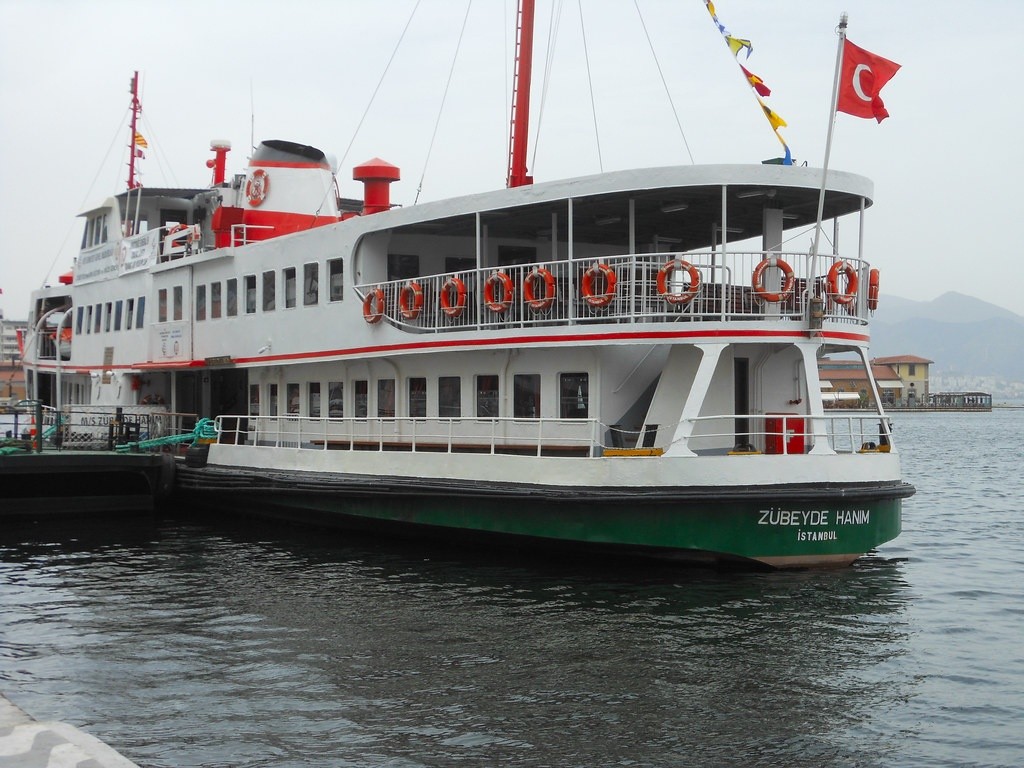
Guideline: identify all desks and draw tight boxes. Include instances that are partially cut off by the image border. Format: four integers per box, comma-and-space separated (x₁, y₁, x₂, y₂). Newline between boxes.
727, 449, 761, 455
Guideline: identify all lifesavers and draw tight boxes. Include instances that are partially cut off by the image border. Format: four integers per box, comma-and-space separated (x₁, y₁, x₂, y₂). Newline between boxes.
440, 278, 466, 317
582, 265, 616, 306
656, 260, 700, 305
869, 269, 880, 310
524, 269, 555, 309
484, 272, 514, 313
362, 288, 385, 325
246, 169, 269, 206
398, 283, 423, 320
170, 224, 193, 246
828, 261, 858, 303
752, 258, 796, 301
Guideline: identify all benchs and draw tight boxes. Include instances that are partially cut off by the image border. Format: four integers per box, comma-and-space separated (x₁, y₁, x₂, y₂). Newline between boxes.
684, 277, 823, 321
535, 268, 657, 323
383, 291, 478, 326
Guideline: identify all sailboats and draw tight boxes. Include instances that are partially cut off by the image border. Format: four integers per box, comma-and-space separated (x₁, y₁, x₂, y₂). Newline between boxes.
17, 0, 916, 567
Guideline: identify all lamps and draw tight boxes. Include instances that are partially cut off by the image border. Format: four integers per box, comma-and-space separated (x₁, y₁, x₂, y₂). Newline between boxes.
661, 203, 689, 213
736, 189, 769, 199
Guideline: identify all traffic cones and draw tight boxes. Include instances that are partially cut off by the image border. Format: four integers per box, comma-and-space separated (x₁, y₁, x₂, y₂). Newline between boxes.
29, 416, 37, 449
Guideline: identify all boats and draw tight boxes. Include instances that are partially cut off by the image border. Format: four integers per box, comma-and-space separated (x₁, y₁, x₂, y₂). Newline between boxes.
45, 312, 72, 359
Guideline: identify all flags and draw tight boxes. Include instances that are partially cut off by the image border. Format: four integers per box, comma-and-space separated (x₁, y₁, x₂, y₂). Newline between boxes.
135, 130, 148, 148
135, 148, 146, 159
837, 38, 902, 124
